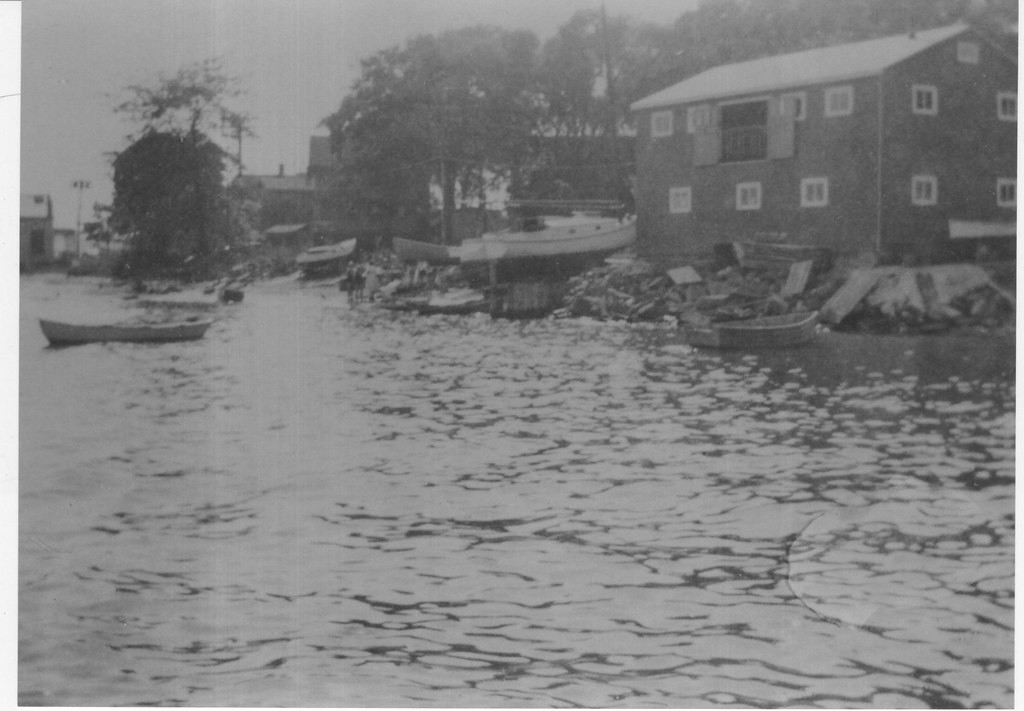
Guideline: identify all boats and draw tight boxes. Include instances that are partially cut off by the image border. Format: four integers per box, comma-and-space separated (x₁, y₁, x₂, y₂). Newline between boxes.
39, 318, 214, 346
692, 310, 820, 348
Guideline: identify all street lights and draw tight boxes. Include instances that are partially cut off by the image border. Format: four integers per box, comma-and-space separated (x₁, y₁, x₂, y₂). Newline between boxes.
74, 180, 90, 261
